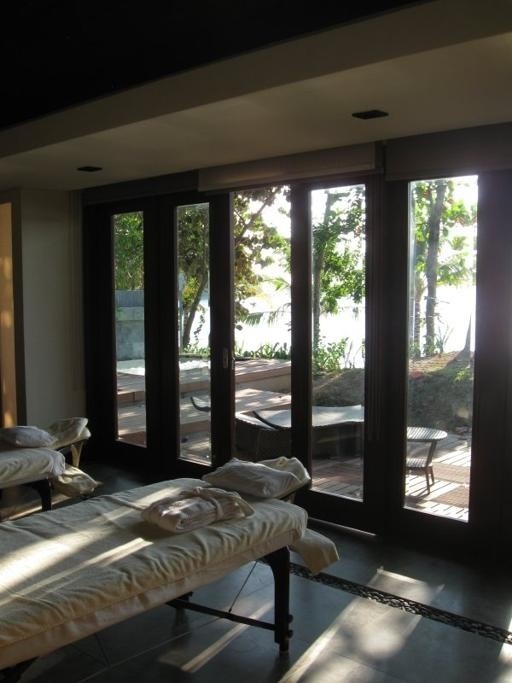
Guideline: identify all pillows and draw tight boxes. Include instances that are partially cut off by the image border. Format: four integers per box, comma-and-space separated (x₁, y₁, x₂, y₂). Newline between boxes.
200, 456, 302, 500
0, 425, 58, 448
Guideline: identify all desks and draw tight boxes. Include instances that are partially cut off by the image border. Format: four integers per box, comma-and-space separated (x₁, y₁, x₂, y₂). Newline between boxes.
406, 426, 448, 495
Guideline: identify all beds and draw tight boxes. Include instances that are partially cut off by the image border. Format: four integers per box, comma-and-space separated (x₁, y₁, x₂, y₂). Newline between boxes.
0, 413, 92, 522
0, 456, 340, 683
